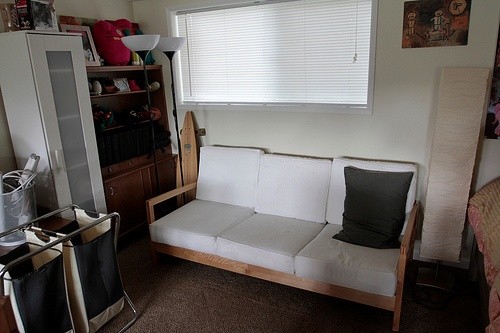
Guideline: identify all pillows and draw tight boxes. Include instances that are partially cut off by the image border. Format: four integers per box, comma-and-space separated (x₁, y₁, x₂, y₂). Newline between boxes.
331, 166, 414, 249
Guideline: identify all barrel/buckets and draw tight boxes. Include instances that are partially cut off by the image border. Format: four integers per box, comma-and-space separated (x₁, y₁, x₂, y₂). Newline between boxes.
0, 183, 38, 244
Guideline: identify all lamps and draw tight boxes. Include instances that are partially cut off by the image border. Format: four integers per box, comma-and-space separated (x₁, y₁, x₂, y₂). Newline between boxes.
121, 33, 185, 213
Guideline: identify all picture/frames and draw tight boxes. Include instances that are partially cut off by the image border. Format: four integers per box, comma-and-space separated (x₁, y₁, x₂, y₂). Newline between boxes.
59, 24, 101, 67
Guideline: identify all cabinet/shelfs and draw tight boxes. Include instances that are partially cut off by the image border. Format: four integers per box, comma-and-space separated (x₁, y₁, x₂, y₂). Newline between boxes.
0, 31, 107, 219
84, 64, 178, 242
0, 205, 88, 333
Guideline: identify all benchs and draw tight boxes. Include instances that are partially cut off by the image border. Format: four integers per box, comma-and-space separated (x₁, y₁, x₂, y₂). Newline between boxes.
145, 145, 422, 332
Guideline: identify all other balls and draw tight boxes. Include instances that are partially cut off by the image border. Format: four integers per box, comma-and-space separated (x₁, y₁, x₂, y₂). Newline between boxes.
92, 81, 102, 93
151, 82, 160, 91
